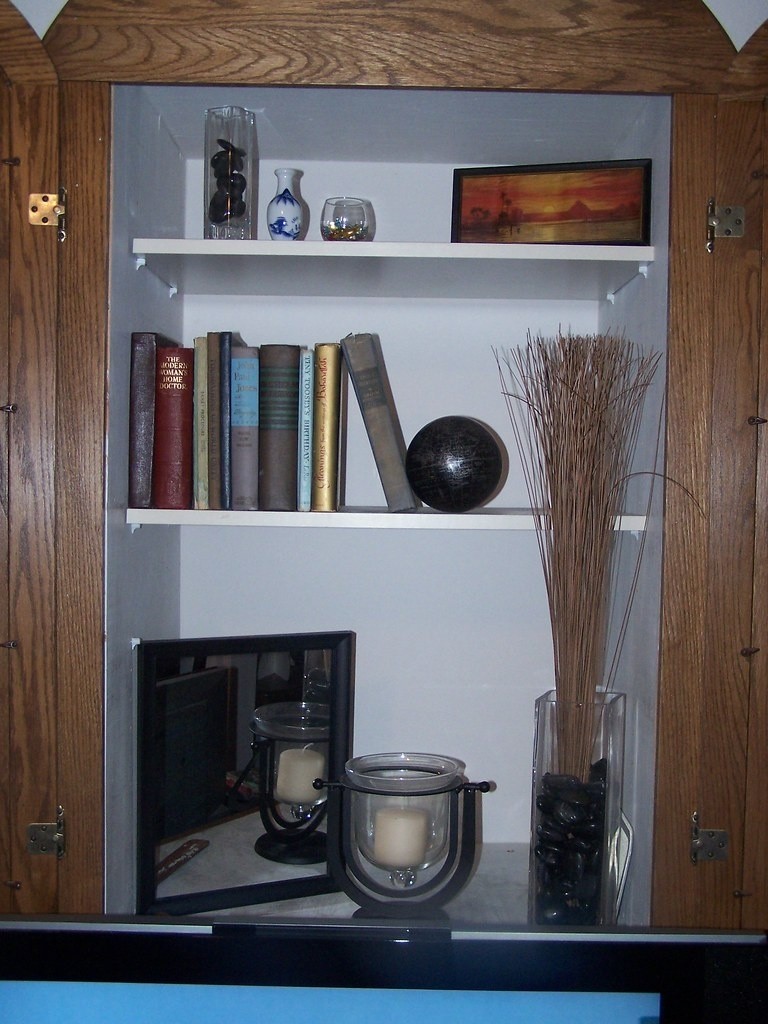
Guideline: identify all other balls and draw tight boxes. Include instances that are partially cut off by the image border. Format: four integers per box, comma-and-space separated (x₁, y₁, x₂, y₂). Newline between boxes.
406, 415, 505, 513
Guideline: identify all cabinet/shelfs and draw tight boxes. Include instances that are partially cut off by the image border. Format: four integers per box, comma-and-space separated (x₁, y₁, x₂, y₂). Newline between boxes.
0, 0, 768, 936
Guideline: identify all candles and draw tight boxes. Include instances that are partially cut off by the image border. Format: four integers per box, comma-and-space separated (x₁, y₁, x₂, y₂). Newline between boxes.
374, 796, 428, 867
277, 743, 326, 804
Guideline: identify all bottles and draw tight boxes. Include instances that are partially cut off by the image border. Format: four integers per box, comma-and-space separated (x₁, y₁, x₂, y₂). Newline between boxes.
267, 167, 303, 240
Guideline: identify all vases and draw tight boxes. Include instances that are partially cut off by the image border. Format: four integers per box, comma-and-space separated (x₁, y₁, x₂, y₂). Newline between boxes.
526, 690, 627, 928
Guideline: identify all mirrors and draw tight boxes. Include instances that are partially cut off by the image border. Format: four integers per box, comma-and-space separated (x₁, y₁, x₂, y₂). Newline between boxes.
129, 629, 358, 917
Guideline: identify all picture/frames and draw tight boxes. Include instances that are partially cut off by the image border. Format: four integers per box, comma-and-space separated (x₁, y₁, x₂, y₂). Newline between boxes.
451, 157, 653, 247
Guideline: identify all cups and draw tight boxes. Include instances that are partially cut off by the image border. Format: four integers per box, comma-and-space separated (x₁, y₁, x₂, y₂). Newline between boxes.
320, 197, 370, 240
203, 106, 256, 240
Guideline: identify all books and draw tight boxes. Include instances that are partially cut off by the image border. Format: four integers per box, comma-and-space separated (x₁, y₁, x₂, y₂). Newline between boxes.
340, 334, 422, 512
129, 331, 347, 513
